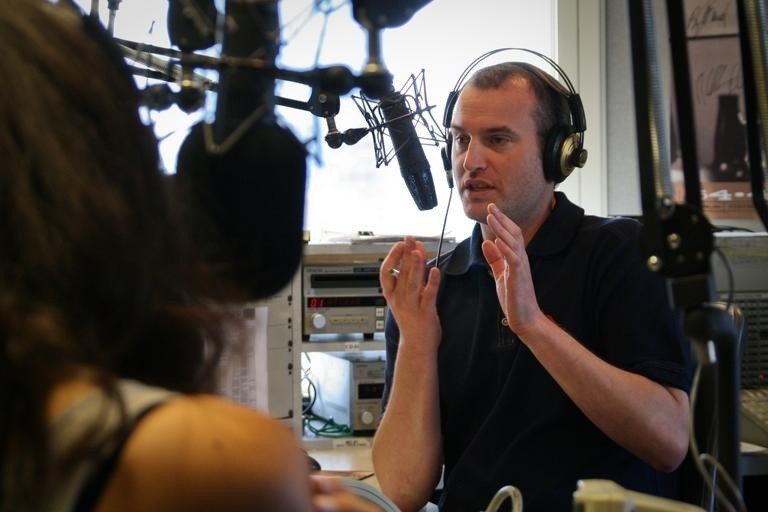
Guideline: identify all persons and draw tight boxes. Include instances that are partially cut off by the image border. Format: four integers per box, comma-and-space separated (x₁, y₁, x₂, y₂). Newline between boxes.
1, 0, 323, 511
367, 58, 700, 511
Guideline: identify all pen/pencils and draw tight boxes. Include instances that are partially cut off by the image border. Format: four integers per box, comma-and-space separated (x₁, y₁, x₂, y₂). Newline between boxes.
388, 268, 400, 277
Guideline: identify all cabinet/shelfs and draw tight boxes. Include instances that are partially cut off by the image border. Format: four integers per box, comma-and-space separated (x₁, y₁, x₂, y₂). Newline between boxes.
295, 248, 455, 455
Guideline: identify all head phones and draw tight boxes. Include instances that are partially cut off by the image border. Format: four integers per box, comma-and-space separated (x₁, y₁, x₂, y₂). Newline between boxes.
440, 47, 588, 189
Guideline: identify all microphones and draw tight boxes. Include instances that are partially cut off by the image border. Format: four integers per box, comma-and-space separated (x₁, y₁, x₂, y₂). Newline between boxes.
174, 0, 309, 308
382, 89, 438, 210
